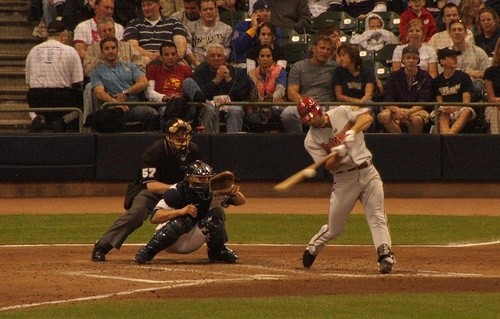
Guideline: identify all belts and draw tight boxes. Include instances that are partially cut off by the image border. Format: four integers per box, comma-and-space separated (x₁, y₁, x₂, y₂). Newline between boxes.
336, 159, 372, 174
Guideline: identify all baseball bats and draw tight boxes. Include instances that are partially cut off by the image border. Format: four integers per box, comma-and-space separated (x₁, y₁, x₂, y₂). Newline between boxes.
270, 150, 339, 194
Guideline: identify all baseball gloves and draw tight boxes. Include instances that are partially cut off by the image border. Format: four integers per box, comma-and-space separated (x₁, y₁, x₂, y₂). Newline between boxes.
208, 171, 240, 198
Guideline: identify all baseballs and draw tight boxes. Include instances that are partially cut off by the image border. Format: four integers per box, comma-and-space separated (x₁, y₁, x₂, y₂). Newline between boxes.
303, 168, 317, 179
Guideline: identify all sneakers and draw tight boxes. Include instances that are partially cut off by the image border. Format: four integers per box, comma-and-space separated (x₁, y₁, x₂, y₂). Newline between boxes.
135, 247, 154, 264
377, 244, 392, 263
207, 245, 238, 263
302, 250, 317, 270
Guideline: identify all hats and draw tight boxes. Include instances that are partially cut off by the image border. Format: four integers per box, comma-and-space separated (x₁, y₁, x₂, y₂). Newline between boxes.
47, 20, 71, 33
251, 1, 271, 15
438, 46, 462, 59
401, 47, 420, 65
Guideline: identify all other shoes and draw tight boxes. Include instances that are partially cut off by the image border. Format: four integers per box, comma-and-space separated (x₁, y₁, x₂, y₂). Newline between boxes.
193, 91, 203, 111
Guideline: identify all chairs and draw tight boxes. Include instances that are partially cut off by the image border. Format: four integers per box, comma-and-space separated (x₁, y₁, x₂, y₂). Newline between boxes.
273, 10, 402, 83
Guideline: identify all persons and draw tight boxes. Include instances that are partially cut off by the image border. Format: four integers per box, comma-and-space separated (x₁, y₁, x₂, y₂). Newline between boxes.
297, 97, 396, 273
24, 0, 500, 135
134, 160, 246, 264
91, 121, 198, 262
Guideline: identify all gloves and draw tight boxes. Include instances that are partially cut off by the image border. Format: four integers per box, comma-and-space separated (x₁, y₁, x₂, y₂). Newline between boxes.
169, 183, 177, 190
331, 144, 347, 157
341, 129, 356, 148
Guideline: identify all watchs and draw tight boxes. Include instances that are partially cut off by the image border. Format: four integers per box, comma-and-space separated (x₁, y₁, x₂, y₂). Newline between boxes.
123, 89, 130, 97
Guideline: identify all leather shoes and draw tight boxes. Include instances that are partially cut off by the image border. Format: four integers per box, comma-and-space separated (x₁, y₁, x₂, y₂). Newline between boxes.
91, 245, 106, 262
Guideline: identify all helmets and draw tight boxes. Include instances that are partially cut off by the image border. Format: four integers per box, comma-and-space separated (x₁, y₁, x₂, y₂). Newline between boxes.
297, 96, 321, 122
163, 116, 193, 157
185, 160, 215, 193
